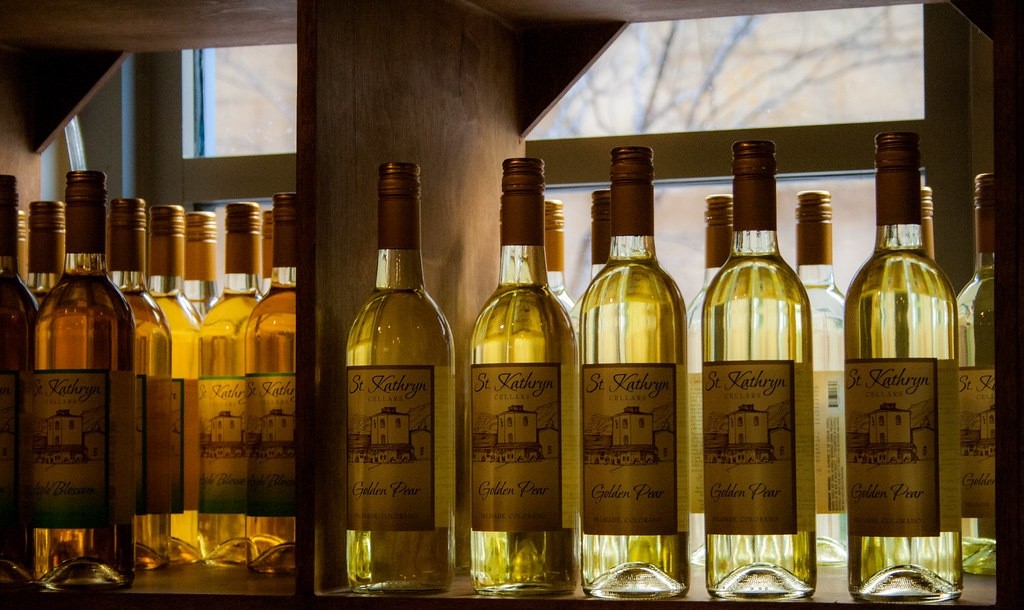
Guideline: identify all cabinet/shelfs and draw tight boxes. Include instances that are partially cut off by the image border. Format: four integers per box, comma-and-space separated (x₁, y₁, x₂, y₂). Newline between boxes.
0, 0, 1024, 610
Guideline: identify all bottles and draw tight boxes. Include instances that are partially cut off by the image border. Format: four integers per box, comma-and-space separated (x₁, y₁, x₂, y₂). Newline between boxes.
0, 168, 295, 588
460, 130, 998, 604
340, 157, 456, 595
